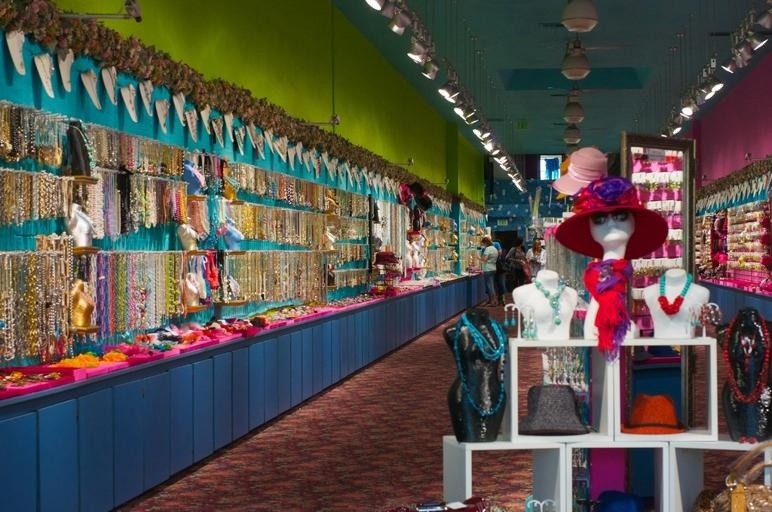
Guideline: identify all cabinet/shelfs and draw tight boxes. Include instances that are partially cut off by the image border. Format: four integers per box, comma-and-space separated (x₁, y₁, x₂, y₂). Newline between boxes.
696, 277, 767, 334
442, 336, 768, 512
1, 269, 488, 507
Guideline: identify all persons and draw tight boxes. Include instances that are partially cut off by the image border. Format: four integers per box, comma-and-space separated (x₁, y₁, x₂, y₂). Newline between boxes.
511, 269, 579, 339
525, 237, 547, 283
442, 306, 506, 443
643, 268, 711, 338
476, 236, 499, 308
544, 158, 560, 180
554, 177, 670, 340
481, 236, 506, 307
716, 307, 772, 442
505, 236, 527, 289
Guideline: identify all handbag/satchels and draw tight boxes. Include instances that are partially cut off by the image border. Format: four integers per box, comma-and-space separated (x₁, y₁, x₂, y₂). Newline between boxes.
694, 441, 772, 512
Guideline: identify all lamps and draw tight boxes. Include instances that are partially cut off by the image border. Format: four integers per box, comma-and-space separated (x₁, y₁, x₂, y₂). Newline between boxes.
482, 68, 491, 139
492, 91, 499, 156
366, 0, 385, 11
500, 110, 513, 171
721, 2, 736, 75
659, 72, 669, 140
454, 17, 467, 118
407, 1, 424, 65
560, 36, 591, 81
391, 1, 405, 35
699, 11, 715, 100
681, 35, 696, 117
465, 26, 474, 118
513, 127, 521, 186
667, 48, 683, 136
735, 1, 752, 61
494, 102, 507, 164
705, 1, 724, 93
560, 1, 597, 33
422, 1, 437, 81
484, 74, 493, 151
473, 50, 481, 139
563, 83, 585, 126
746, 1, 768, 51
449, 8, 459, 101
563, 125, 582, 146
439, 1, 451, 98
508, 117, 518, 178
467, 36, 477, 126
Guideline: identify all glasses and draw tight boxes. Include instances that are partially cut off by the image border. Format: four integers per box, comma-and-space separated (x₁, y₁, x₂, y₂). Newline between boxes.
590, 209, 628, 224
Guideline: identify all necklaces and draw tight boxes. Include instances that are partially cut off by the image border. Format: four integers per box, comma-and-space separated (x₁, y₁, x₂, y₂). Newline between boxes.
1, 230, 326, 364
722, 315, 771, 405
454, 311, 506, 416
324, 243, 368, 268
531, 278, 566, 326
407, 211, 489, 284
225, 198, 324, 252
74, 119, 186, 178
325, 186, 370, 218
72, 166, 210, 242
658, 273, 693, 315
324, 214, 369, 243
0, 171, 77, 250
0, 99, 72, 167
185, 148, 325, 212
325, 267, 371, 291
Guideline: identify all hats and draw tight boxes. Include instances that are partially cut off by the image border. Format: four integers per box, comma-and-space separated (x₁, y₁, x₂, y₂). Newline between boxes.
552, 147, 608, 196
374, 252, 398, 265
520, 385, 588, 433
622, 394, 686, 433
407, 182, 424, 195
556, 176, 668, 260
414, 195, 432, 211
